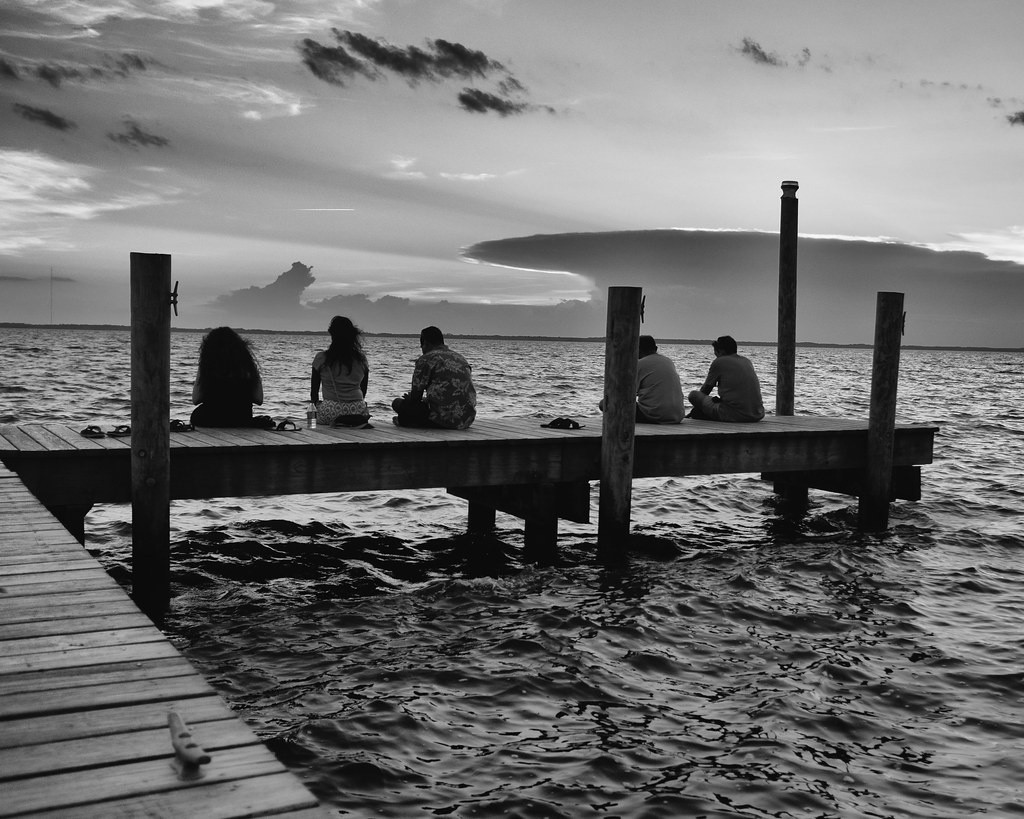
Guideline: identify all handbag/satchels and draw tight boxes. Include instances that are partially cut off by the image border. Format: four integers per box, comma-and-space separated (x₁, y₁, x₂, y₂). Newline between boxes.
332, 414, 373, 428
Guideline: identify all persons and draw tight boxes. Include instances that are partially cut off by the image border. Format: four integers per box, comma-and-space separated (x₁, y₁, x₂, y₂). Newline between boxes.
392, 326, 477, 430
684, 335, 765, 423
599, 335, 685, 424
310, 316, 369, 425
190, 326, 264, 428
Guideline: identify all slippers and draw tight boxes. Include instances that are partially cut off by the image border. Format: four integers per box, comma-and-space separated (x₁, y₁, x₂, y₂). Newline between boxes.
540, 417, 585, 429
169, 420, 195, 432
80, 425, 105, 438
106, 425, 131, 436
271, 420, 302, 431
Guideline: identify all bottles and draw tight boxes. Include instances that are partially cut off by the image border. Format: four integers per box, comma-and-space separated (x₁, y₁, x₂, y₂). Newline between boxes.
307, 400, 317, 430
